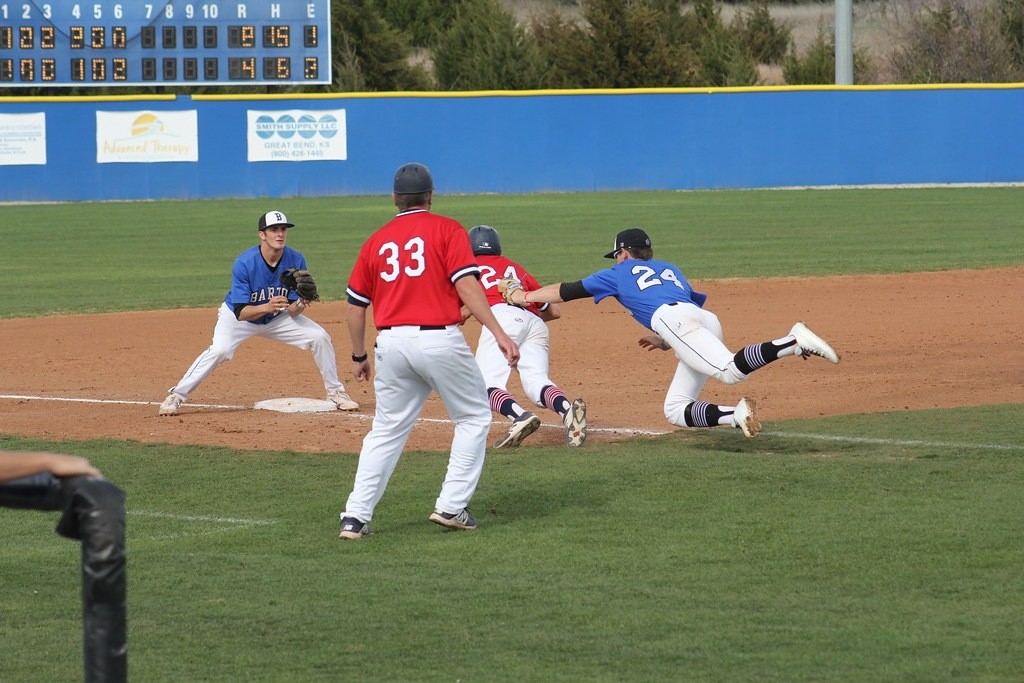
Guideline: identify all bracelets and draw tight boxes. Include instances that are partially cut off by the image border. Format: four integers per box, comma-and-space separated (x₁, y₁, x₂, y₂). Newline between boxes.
297, 298, 308, 308
524, 291, 530, 303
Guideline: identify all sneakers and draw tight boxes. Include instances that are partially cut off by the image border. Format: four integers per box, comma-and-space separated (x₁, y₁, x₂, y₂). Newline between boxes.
564, 397, 587, 447
732, 396, 762, 438
158, 395, 182, 416
788, 321, 839, 364
339, 516, 368, 540
428, 506, 477, 529
332, 392, 359, 412
493, 411, 541, 449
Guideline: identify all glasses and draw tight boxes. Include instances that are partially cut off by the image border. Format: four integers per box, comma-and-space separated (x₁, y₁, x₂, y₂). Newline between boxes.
613, 246, 632, 259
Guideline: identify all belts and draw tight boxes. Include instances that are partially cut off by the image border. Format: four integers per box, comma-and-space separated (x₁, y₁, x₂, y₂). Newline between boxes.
508, 302, 524, 311
668, 302, 678, 306
380, 324, 446, 330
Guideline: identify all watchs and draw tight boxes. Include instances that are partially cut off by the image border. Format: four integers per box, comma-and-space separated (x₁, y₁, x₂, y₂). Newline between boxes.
352, 351, 368, 362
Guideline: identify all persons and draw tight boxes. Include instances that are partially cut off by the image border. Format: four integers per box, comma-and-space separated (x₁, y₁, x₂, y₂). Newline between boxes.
459, 225, 587, 448
157, 210, 359, 416
497, 228, 839, 437
0, 449, 103, 485
337, 162, 520, 539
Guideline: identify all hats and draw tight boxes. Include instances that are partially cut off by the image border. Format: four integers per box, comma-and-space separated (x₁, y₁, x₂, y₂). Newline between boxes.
259, 211, 295, 232
604, 228, 652, 259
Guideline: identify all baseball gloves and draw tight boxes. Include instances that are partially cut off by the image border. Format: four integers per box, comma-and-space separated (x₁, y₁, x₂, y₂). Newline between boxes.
497, 277, 530, 308
279, 268, 319, 302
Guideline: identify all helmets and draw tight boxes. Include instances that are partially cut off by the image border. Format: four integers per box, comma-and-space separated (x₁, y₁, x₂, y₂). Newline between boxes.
468, 225, 502, 256
393, 163, 435, 193
